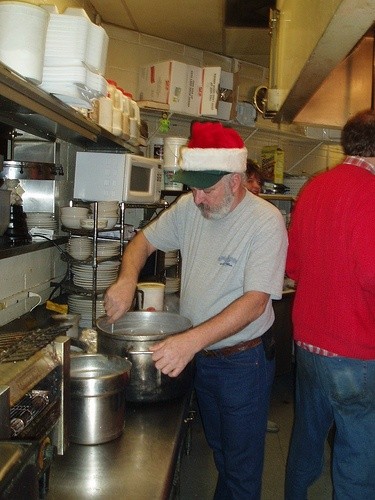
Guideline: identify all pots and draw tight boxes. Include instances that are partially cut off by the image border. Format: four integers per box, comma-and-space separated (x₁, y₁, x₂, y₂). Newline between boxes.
96, 312, 192, 403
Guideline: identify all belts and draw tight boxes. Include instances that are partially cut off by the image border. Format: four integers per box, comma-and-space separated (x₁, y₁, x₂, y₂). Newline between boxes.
198, 337, 263, 357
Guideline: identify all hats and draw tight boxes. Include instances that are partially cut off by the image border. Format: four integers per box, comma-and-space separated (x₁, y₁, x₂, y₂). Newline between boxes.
171, 120, 247, 188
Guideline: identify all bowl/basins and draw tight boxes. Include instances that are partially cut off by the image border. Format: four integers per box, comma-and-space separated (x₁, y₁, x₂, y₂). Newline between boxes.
59, 201, 120, 231
67, 236, 93, 260
16, 4, 110, 109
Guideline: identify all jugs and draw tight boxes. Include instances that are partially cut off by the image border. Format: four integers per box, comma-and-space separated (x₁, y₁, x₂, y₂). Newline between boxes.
136, 282, 165, 311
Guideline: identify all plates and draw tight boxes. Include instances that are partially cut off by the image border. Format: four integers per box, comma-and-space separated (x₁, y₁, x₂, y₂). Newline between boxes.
91, 240, 121, 258
26, 213, 58, 233
0, 0, 50, 85
164, 250, 180, 293
67, 260, 121, 323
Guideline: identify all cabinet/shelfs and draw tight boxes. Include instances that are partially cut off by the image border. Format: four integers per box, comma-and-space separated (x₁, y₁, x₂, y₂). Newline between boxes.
67, 189, 340, 328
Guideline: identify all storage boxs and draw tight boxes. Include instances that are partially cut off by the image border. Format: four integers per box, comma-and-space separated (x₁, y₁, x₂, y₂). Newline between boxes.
200, 66, 233, 120
137, 60, 201, 112
260, 146, 285, 184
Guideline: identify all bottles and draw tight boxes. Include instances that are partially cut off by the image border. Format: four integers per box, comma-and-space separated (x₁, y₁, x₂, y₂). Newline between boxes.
79, 79, 140, 147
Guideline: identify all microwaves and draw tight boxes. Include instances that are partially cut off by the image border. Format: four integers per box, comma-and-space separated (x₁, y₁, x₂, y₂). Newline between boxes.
72, 151, 163, 205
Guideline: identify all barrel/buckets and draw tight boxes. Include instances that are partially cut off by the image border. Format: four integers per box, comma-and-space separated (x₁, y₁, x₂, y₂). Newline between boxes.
52, 313, 79, 338
146, 136, 189, 191
68, 354, 132, 445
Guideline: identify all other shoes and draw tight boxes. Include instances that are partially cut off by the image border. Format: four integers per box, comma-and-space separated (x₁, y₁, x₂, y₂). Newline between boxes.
267, 420, 278, 432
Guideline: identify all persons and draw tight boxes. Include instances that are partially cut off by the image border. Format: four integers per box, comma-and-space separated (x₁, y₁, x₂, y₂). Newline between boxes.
246, 157, 280, 432
286, 107, 375, 500
102, 122, 289, 500
